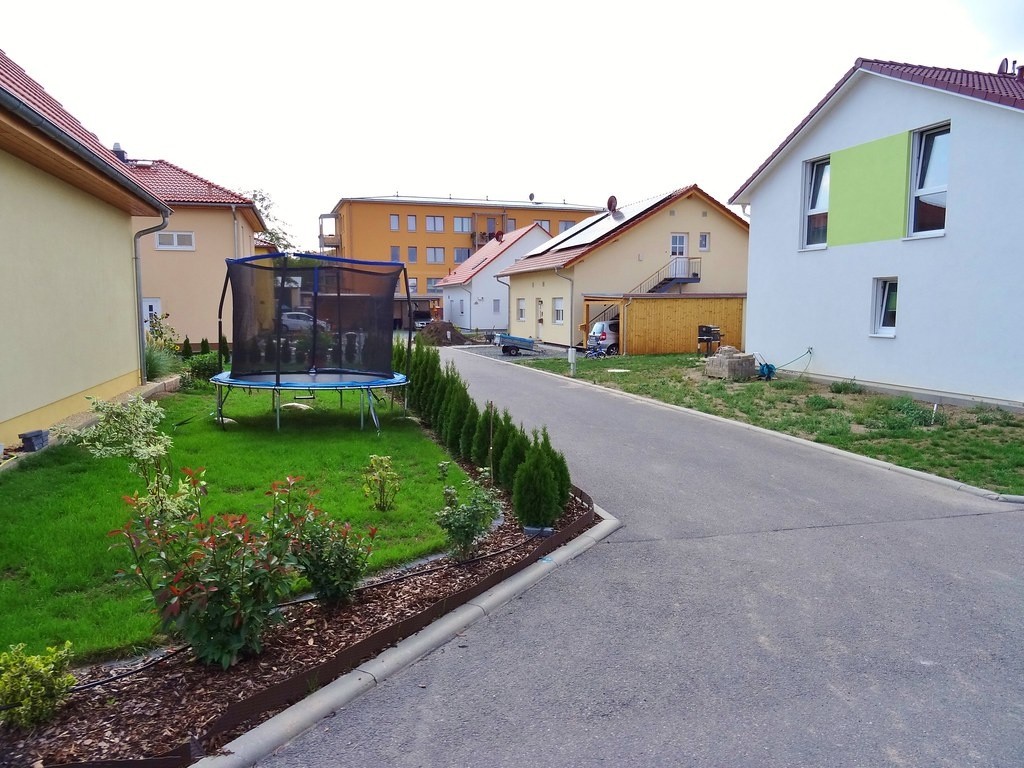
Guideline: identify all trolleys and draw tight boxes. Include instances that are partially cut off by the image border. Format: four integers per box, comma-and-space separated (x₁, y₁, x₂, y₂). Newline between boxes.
493, 333, 545, 356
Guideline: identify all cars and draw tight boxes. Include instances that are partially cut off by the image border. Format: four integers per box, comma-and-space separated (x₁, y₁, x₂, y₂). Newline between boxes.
279, 312, 330, 335
586, 320, 620, 355
413, 310, 435, 330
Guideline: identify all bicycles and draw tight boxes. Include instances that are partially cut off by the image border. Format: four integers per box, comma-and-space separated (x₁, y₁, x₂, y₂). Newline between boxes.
584, 345, 606, 358
